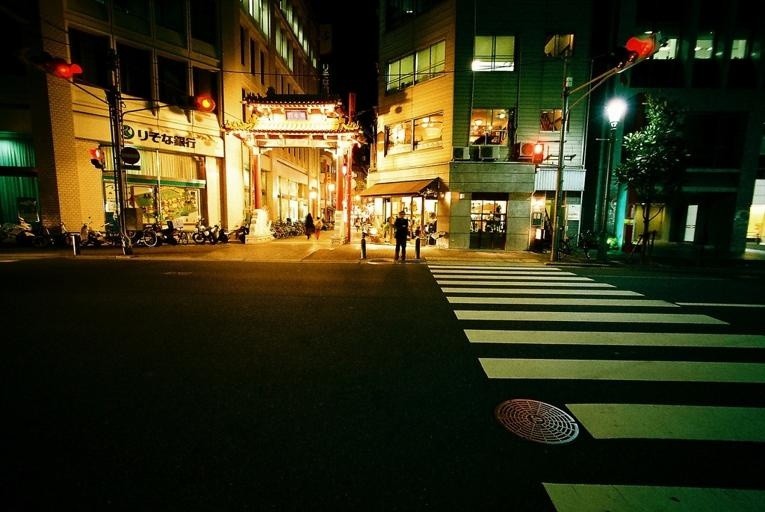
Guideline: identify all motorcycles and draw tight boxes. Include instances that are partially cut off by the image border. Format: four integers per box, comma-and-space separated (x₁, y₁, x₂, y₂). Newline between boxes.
80, 218, 219, 248
0, 201, 72, 248
237, 221, 305, 243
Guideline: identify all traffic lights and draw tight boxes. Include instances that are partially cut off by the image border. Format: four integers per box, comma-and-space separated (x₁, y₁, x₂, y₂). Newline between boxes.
91, 147, 105, 168
534, 143, 544, 163
49, 60, 83, 78
616, 41, 653, 69
178, 94, 216, 112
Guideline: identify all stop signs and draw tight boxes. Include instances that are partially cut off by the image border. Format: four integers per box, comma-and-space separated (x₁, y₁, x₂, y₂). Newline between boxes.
121, 147, 140, 164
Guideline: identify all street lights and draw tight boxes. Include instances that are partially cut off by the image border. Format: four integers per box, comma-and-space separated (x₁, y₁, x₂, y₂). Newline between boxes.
603, 98, 628, 231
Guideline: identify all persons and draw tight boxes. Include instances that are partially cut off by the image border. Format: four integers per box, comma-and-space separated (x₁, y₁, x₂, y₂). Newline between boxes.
489, 205, 502, 221
355, 216, 363, 233
383, 212, 437, 245
305, 213, 323, 240
394, 211, 408, 263
